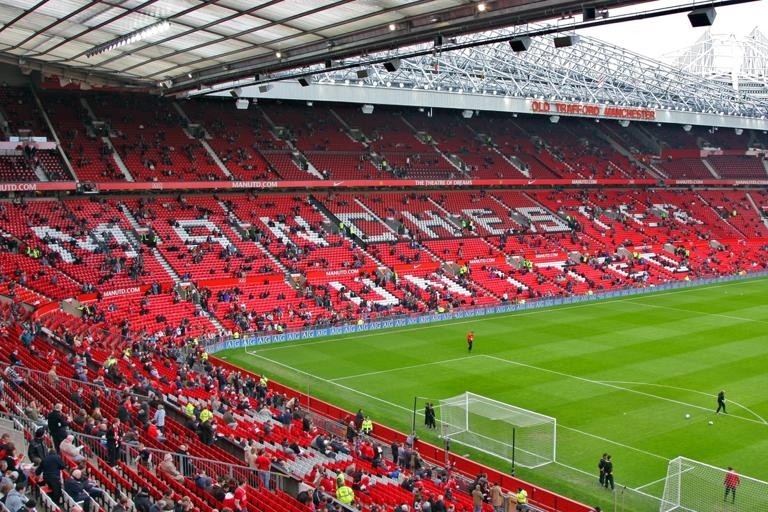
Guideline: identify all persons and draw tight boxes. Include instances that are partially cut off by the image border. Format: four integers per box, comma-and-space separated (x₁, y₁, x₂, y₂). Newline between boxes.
722, 466, 740, 504
440, 460, 531, 512
467, 331, 475, 352
209, 360, 439, 511
603, 455, 616, 491
0, 340, 208, 512
1, 83, 419, 341
588, 506, 603, 511
715, 389, 726, 415
420, 112, 767, 315
597, 453, 607, 485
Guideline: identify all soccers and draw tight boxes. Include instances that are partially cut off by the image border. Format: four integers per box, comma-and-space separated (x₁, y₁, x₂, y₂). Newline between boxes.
709, 421, 713, 425
685, 414, 690, 419
